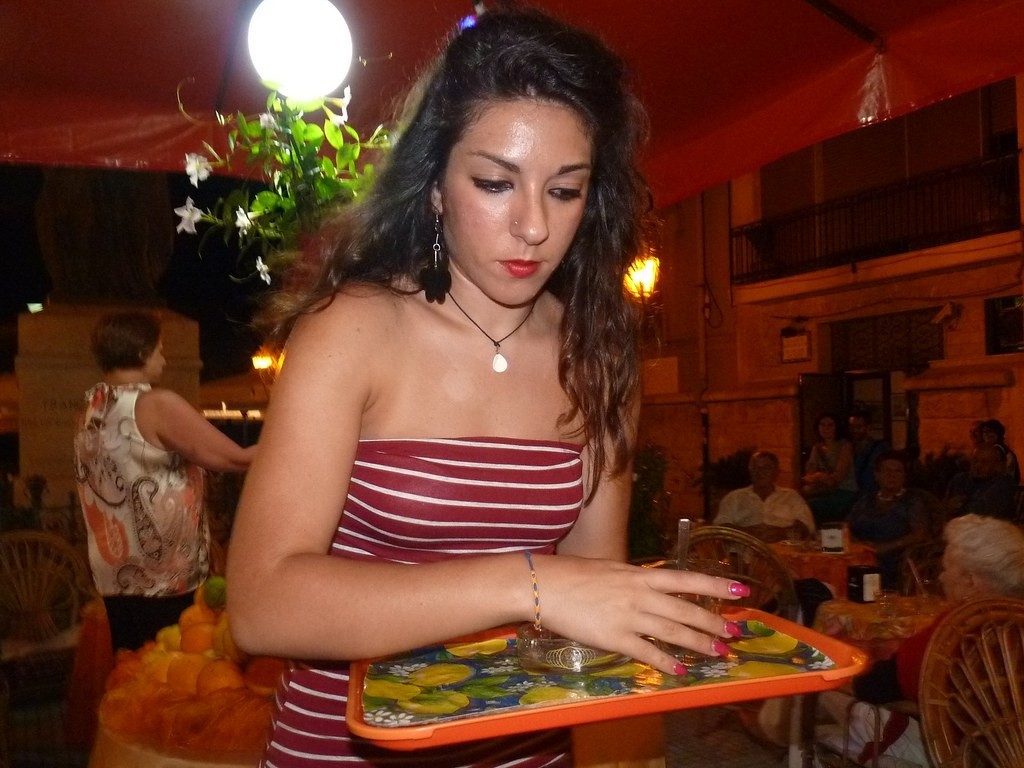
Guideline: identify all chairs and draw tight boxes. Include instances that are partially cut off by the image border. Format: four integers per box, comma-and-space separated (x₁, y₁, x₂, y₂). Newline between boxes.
0, 530, 91, 739
917, 594, 1024, 768
677, 519, 798, 621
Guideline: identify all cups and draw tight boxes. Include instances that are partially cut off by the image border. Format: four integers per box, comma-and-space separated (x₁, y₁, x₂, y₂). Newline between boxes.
640, 559, 725, 667
915, 579, 940, 614
872, 589, 900, 618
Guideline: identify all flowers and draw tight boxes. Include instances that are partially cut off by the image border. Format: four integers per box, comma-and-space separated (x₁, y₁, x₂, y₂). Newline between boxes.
157, 55, 400, 288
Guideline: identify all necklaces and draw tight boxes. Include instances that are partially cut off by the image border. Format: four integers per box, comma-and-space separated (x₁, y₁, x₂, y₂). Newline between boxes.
435, 292, 541, 374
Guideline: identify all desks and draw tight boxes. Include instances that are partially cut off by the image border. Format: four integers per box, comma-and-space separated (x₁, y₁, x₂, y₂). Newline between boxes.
751, 538, 881, 601
811, 595, 954, 702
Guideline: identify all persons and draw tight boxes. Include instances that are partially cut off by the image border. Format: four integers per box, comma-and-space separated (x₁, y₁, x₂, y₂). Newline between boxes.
713, 413, 1024, 622
226, 8, 750, 768
72, 311, 262, 659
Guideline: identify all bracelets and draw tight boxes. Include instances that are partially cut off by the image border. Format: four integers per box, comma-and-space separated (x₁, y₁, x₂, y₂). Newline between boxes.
523, 549, 544, 634
